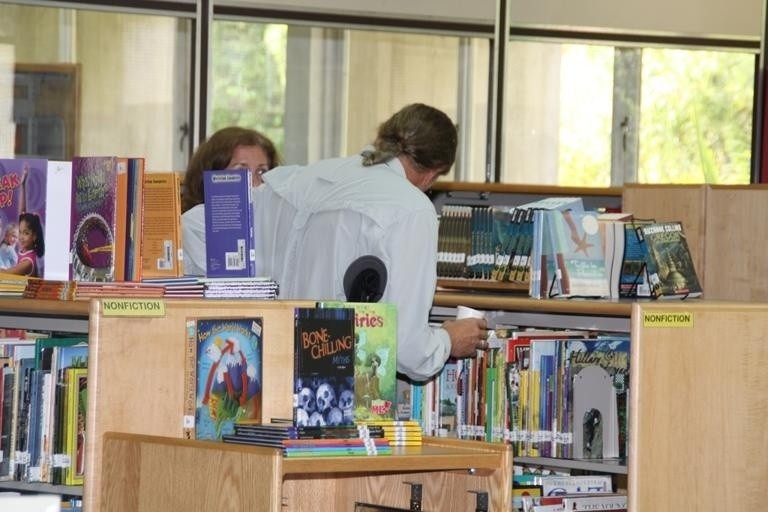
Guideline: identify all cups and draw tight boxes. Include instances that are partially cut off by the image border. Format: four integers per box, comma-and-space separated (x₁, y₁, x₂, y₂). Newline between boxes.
455, 305, 487, 322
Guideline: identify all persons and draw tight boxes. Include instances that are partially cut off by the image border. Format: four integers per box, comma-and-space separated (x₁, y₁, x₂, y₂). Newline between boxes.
250, 103, 490, 387
176, 125, 280, 276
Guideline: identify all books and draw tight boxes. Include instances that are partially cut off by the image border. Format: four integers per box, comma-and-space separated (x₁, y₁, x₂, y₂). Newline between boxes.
1, 156, 279, 299
397, 323, 630, 458
1, 328, 88, 512
436, 197, 701, 301
183, 302, 421, 457
512, 463, 627, 512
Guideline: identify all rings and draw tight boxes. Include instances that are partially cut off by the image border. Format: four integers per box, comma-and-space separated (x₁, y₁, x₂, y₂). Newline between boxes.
478, 339, 486, 348
